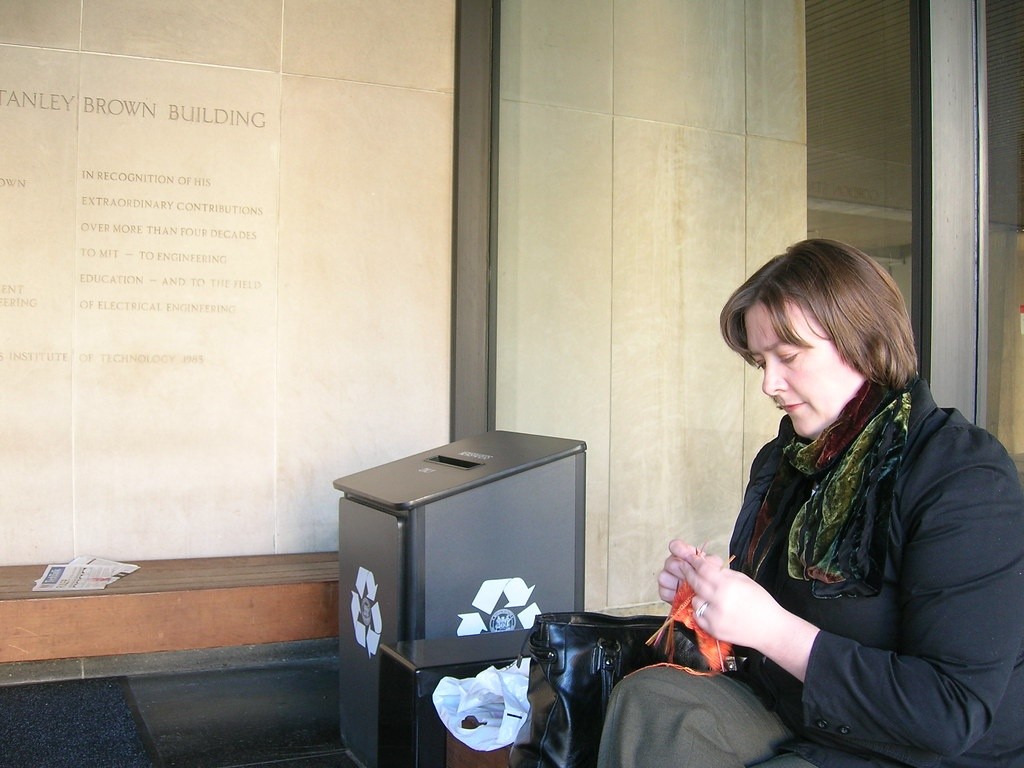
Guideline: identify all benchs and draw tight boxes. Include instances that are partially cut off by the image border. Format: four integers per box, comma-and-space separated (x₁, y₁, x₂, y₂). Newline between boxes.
377, 629, 533, 768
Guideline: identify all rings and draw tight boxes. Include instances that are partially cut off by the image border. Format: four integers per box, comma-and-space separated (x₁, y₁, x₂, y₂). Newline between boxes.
697, 602, 709, 616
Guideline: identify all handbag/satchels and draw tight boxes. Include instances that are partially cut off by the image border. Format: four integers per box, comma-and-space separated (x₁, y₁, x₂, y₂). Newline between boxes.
432, 658, 531, 753
507, 611, 738, 768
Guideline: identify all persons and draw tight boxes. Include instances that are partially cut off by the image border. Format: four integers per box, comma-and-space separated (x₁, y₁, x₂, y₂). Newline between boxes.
595, 237, 1024, 768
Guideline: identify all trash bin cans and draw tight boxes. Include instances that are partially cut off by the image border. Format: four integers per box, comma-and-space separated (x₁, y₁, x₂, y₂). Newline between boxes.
332, 429, 589, 768
